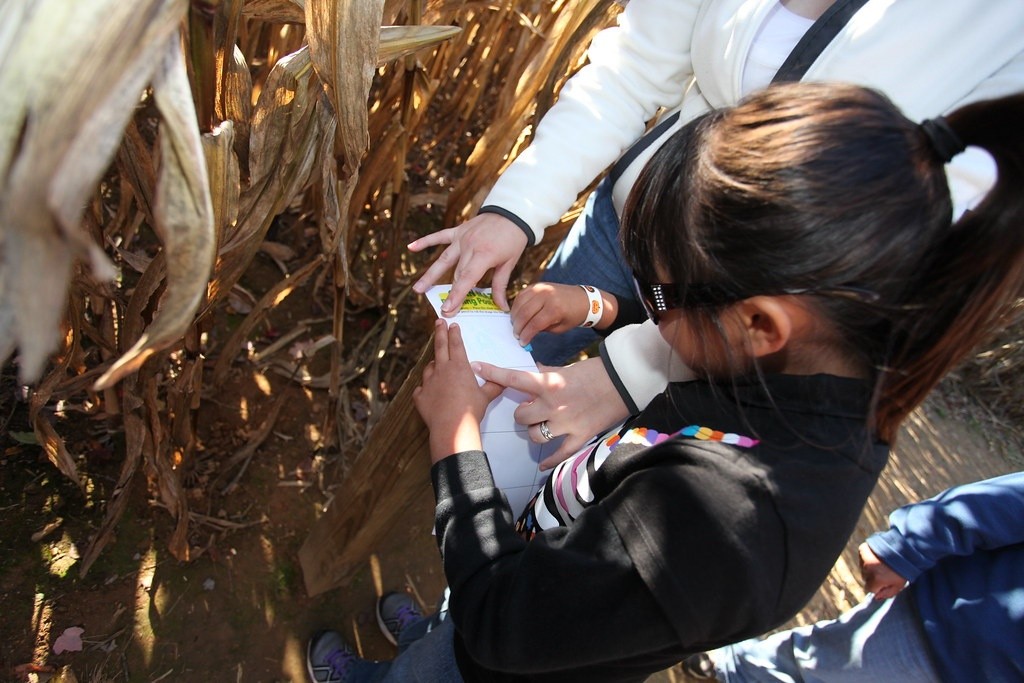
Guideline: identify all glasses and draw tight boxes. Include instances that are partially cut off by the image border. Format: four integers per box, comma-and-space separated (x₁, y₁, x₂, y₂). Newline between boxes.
631, 267, 880, 326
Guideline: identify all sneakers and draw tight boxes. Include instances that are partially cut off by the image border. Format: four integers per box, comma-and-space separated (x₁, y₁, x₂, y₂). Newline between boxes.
376, 591, 425, 648
682, 652, 715, 680
306, 628, 358, 683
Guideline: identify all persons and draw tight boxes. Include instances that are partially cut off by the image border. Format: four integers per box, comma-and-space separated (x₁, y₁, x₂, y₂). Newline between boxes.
310, 0, 1023, 683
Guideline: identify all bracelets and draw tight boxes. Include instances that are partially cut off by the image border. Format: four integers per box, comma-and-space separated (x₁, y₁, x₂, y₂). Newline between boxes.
578, 284, 604, 329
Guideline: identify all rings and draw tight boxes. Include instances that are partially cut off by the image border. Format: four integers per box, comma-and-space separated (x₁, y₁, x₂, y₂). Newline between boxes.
540, 421, 555, 441
